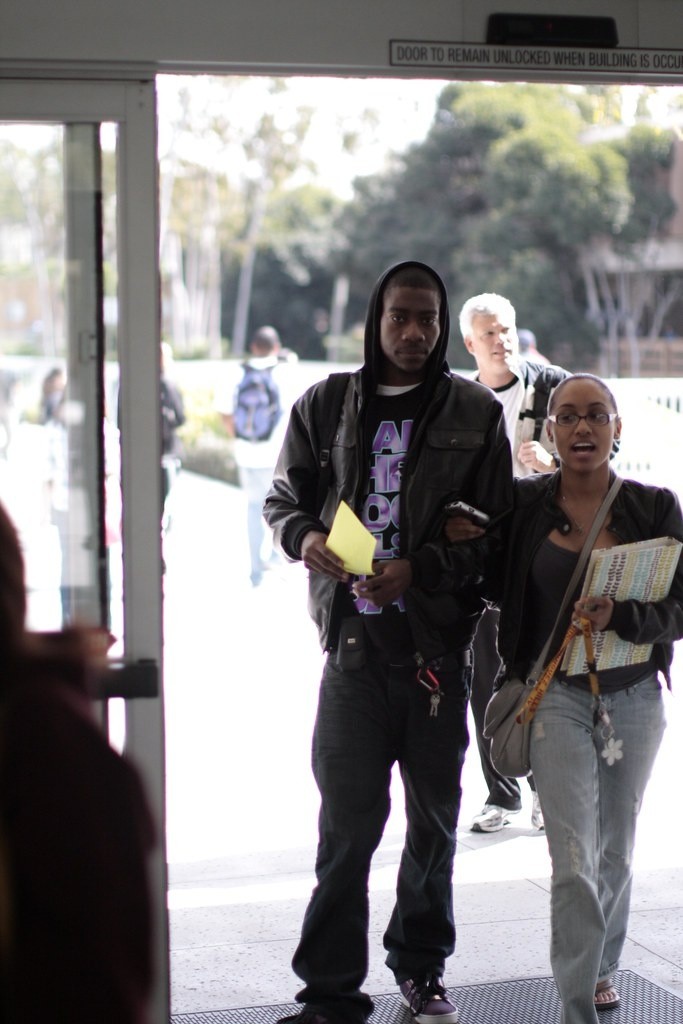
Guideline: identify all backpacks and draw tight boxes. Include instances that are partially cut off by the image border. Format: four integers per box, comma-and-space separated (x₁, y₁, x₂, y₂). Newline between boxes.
233, 361, 281, 441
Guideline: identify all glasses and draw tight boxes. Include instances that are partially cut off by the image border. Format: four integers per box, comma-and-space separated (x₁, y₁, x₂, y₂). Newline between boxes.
547, 411, 618, 428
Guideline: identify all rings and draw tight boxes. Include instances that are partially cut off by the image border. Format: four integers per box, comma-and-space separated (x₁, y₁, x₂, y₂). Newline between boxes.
572, 620, 580, 626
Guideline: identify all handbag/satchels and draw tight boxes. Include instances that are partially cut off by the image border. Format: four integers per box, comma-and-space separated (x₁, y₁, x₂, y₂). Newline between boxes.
336, 616, 369, 672
488, 679, 535, 777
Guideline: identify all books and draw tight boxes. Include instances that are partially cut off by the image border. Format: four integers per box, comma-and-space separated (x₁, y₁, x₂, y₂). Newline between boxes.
561, 536, 683, 676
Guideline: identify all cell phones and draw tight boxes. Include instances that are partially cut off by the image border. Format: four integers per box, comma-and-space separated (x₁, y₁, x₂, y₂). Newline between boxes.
446, 501, 489, 527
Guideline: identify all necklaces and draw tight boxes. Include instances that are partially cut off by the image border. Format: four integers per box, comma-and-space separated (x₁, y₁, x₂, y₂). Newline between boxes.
560, 494, 601, 535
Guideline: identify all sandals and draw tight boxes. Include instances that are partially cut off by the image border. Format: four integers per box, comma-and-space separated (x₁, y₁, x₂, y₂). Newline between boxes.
594, 976, 620, 1009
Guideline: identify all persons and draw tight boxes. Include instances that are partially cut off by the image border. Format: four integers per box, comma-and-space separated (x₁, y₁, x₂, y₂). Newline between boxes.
221, 325, 300, 588
449, 372, 683, 1024
0, 338, 184, 1024
460, 292, 577, 833
516, 329, 550, 366
263, 262, 514, 1024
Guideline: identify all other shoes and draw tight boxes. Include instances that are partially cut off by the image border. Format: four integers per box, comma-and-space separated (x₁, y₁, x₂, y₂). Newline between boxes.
274, 995, 339, 1024
397, 970, 460, 1024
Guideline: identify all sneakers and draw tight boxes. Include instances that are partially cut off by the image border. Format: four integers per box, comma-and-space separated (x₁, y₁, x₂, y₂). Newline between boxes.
470, 803, 520, 833
531, 791, 547, 829
